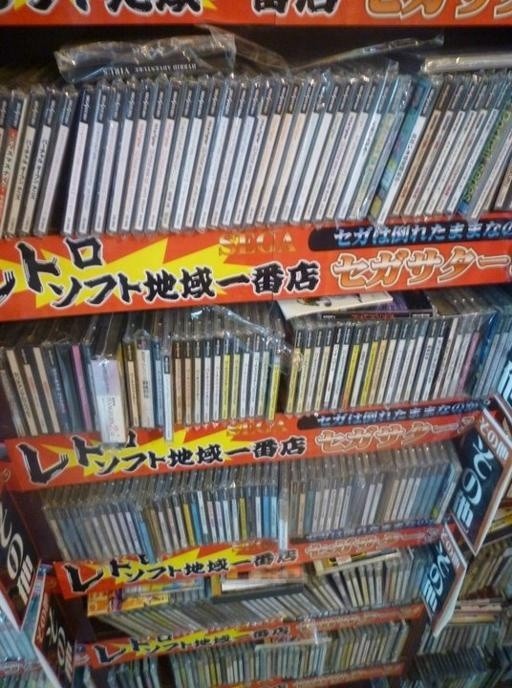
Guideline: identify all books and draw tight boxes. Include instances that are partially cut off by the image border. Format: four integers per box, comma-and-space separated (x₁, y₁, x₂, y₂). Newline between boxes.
0, 498, 80, 688
88, 546, 439, 639
445, 398, 512, 551
34, 440, 466, 560
0, 283, 512, 439
79, 619, 424, 688
416, 539, 509, 625
0, 35, 512, 234
415, 610, 512, 686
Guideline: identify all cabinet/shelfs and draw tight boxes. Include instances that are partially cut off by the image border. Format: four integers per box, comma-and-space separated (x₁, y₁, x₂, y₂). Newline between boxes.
0, 0, 512, 688
